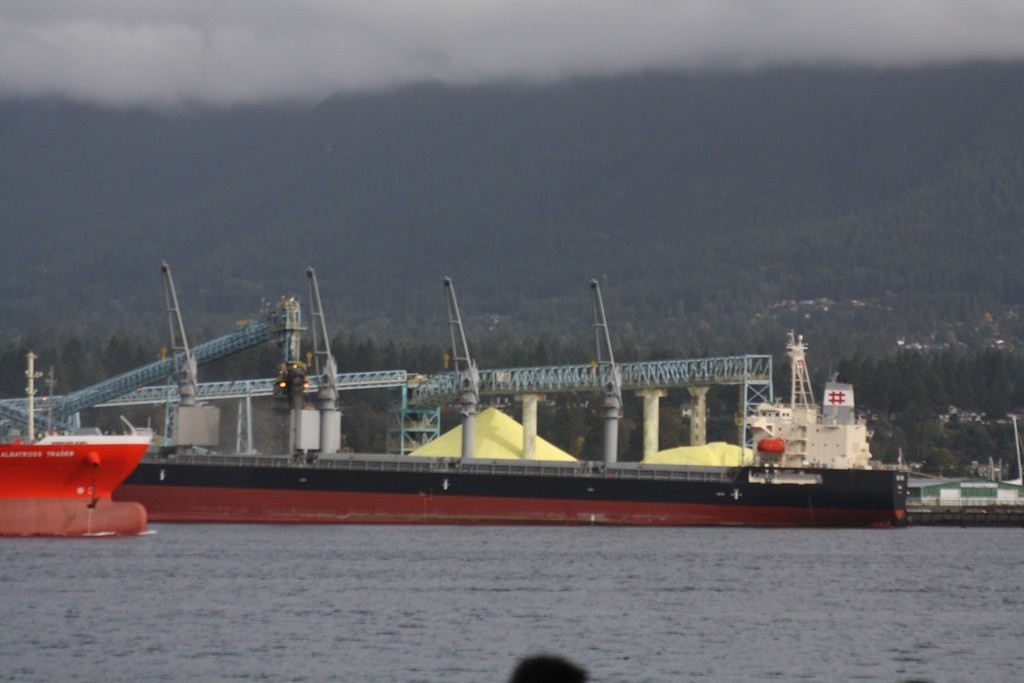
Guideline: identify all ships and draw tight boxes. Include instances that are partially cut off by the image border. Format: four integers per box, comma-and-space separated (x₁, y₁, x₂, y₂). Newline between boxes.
116, 257, 911, 533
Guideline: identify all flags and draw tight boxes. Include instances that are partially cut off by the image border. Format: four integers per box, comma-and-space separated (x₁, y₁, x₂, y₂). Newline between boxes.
897, 456, 901, 464
797, 360, 804, 368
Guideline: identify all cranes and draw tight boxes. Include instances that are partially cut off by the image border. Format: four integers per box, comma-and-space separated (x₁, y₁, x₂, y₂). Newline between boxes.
1, 300, 411, 440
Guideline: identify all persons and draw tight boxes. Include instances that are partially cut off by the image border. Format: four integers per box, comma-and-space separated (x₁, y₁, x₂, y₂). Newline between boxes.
763, 459, 770, 473
507, 655, 589, 682
30, 431, 42, 444
61, 426, 67, 435
772, 459, 779, 472
104, 429, 127, 434
50, 425, 58, 435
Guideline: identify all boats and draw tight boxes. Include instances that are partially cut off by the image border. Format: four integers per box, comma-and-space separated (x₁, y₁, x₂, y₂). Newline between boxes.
0, 352, 152, 535
